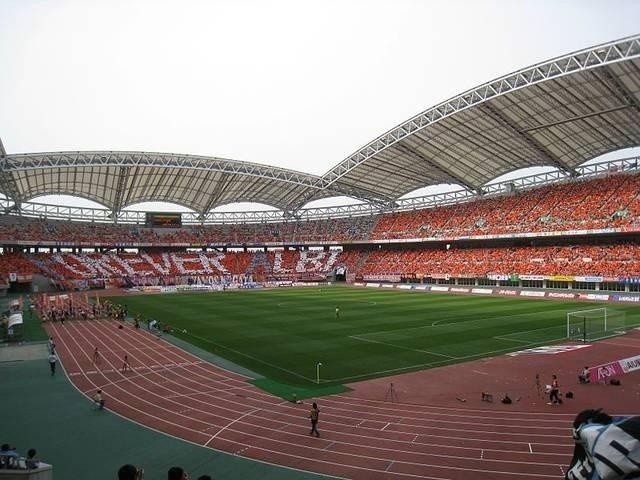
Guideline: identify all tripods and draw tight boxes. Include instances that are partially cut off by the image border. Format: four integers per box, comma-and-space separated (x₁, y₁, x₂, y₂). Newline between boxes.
528, 380, 544, 400
384, 385, 399, 404
122, 359, 130, 371
92, 351, 101, 365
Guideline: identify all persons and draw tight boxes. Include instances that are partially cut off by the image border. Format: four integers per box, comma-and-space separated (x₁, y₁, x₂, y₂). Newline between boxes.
0, 443, 39, 470
48, 351, 58, 377
93, 389, 106, 409
334, 306, 341, 321
0, 292, 188, 346
198, 474, 212, 480
92, 347, 99, 364
166, 466, 191, 480
578, 366, 590, 385
118, 462, 145, 480
122, 355, 129, 374
547, 374, 563, 406
570, 327, 574, 336
307, 402, 321, 438
341, 167, 637, 303
0, 207, 344, 300
50, 343, 55, 356
